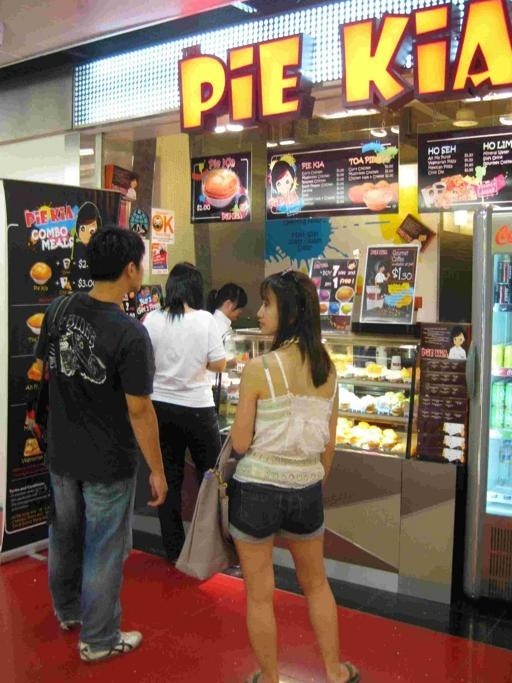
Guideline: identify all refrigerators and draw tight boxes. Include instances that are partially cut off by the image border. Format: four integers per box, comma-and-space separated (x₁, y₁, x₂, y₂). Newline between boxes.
463, 204, 511, 605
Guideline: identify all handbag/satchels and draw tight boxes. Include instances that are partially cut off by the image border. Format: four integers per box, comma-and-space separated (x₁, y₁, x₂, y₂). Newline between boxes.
32, 383, 47, 448
174, 432, 239, 580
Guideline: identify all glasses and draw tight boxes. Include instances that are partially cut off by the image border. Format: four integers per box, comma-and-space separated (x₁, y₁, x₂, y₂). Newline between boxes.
281, 267, 300, 292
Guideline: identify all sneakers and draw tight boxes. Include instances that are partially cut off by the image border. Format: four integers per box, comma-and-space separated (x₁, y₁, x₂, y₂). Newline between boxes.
79, 631, 142, 662
60, 619, 82, 629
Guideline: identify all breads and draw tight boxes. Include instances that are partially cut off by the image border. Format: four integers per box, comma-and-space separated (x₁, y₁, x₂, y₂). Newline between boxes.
30, 262, 51, 284
30, 313, 45, 328
202, 169, 239, 208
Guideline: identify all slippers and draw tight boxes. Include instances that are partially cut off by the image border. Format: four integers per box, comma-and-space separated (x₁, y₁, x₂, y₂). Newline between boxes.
340, 662, 360, 682
247, 671, 261, 682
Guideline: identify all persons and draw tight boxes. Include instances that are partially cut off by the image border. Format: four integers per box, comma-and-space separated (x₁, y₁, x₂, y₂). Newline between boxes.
447, 325, 468, 360
237, 196, 249, 220
75, 200, 102, 246
207, 280, 248, 389
142, 259, 242, 578
32, 220, 170, 662
269, 160, 300, 212
373, 262, 391, 294
126, 173, 138, 199
226, 267, 363, 681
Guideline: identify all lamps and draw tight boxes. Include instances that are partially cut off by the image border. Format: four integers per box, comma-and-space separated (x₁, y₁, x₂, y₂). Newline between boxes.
212, 114, 248, 135
498, 99, 512, 125
451, 100, 480, 127
369, 109, 401, 137
267, 123, 299, 148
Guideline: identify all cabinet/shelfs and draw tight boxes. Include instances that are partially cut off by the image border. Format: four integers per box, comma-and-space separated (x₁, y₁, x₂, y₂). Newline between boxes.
212, 329, 422, 460
